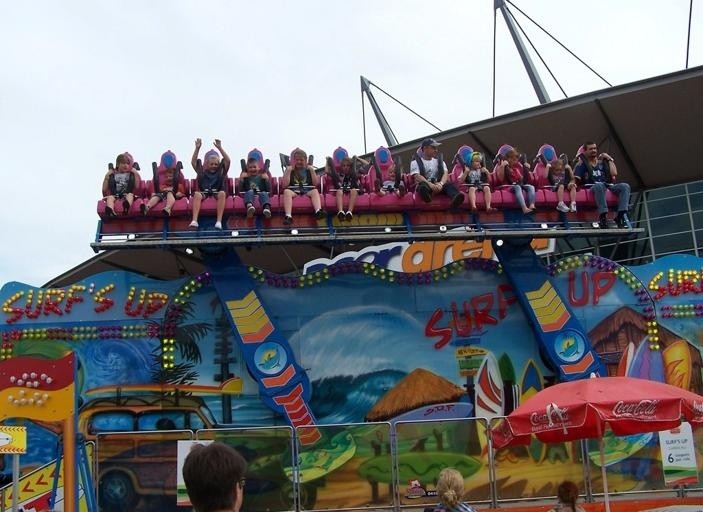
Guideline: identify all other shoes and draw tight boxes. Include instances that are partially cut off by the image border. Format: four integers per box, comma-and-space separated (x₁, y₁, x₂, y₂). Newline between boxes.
487, 208, 498, 215
345, 211, 352, 222
283, 216, 293, 225
373, 179, 381, 192
523, 209, 533, 215
337, 211, 345, 222
471, 206, 477, 214
105, 206, 117, 218
247, 206, 255, 219
263, 208, 271, 218
419, 186, 432, 203
598, 214, 609, 229
399, 184, 405, 198
613, 214, 630, 229
315, 209, 328, 220
215, 221, 222, 230
529, 207, 536, 215
122, 200, 130, 215
450, 193, 465, 211
188, 220, 198, 228
139, 203, 148, 216
162, 207, 171, 217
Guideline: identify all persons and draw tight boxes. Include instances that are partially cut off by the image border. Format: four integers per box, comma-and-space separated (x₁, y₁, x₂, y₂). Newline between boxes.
547, 480, 586, 512
182, 442, 247, 512
424, 468, 477, 512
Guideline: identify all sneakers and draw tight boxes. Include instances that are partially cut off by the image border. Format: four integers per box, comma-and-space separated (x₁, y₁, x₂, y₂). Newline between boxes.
556, 203, 571, 213
569, 202, 577, 213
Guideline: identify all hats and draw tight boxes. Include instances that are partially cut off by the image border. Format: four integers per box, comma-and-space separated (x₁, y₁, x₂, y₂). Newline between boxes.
418, 138, 441, 148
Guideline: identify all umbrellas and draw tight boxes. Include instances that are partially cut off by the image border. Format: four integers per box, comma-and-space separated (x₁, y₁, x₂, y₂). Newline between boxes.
489, 372, 703, 512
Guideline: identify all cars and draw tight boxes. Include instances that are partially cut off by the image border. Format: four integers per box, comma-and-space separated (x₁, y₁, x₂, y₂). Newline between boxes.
79, 397, 325, 510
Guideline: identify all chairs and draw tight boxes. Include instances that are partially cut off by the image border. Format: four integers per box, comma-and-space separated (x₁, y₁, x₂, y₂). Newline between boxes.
97, 156, 622, 233
157, 418, 176, 430
